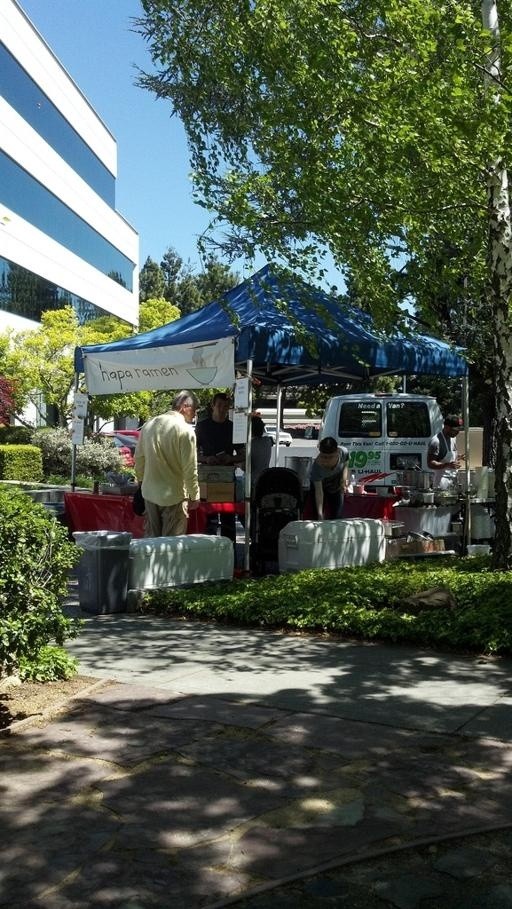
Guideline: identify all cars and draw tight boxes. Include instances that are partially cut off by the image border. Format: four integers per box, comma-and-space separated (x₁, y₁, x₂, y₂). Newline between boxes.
98, 428, 137, 465
262, 424, 291, 445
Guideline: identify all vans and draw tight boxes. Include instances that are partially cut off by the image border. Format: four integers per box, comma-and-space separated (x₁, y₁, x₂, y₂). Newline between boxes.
317, 390, 459, 490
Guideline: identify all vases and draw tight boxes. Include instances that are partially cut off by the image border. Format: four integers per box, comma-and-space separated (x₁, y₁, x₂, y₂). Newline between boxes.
100, 484, 138, 495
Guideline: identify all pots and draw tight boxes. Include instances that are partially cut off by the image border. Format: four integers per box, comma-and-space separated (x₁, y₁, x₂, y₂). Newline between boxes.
396, 465, 434, 490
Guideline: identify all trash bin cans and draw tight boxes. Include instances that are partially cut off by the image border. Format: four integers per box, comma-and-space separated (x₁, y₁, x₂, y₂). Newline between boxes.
72, 530, 133, 615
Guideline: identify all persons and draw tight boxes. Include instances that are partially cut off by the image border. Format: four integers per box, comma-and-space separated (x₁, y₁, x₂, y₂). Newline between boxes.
427, 414, 465, 491
195, 393, 246, 542
308, 437, 352, 520
134, 388, 201, 537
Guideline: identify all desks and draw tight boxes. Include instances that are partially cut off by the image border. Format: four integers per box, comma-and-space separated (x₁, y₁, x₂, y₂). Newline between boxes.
303, 493, 413, 522
64, 491, 245, 536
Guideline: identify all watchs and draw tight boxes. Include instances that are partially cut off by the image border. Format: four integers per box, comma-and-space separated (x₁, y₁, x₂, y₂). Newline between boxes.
237, 416, 274, 542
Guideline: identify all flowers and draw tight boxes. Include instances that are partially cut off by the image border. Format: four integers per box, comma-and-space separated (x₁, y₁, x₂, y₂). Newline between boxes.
103, 471, 133, 487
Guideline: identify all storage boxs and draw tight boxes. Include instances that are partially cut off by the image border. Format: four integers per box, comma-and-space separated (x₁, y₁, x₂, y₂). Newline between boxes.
197, 465, 236, 503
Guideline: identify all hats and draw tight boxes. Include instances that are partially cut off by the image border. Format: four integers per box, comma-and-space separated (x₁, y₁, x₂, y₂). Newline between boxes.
319, 436, 338, 454
443, 416, 464, 431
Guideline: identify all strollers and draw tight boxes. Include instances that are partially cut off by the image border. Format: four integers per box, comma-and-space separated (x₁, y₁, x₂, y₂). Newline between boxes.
251, 465, 303, 568
